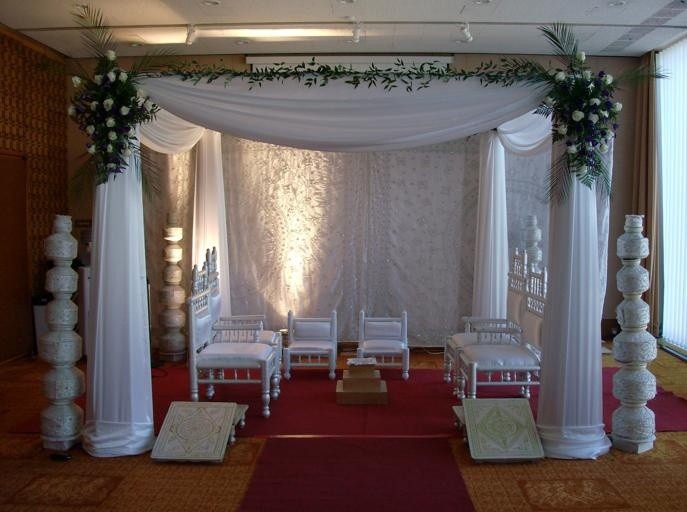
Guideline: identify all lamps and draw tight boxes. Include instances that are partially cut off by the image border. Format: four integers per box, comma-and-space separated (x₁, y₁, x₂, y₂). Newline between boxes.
178, 23, 473, 46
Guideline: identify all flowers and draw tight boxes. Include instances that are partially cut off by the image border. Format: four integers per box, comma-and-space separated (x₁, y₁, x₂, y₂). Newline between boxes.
531, 23, 666, 202
65, 5, 163, 185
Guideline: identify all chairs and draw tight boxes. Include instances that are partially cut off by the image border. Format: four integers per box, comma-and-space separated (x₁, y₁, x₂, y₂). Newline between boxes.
356, 310, 409, 380
444, 247, 528, 384
458, 264, 546, 399
283, 311, 339, 380
187, 262, 279, 417
207, 247, 282, 394
